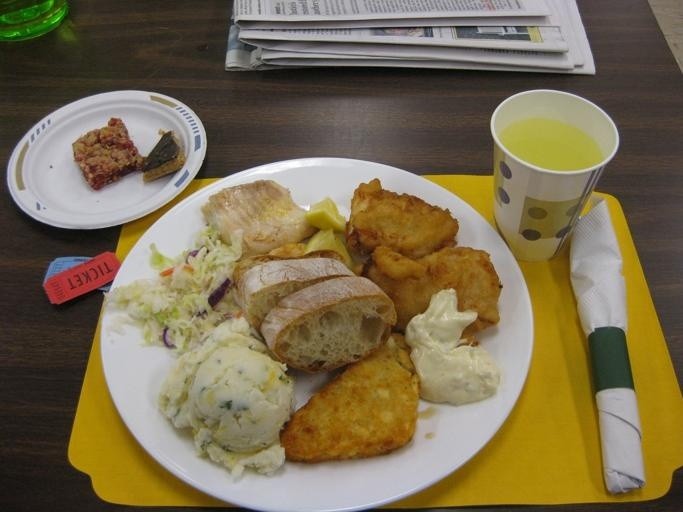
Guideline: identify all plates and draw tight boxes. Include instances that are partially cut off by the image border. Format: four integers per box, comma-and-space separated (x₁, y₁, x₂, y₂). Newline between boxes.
100, 154, 537, 511
6, 89, 207, 230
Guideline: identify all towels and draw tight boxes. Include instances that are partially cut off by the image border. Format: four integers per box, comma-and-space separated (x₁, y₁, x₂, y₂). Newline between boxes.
569, 190, 644, 494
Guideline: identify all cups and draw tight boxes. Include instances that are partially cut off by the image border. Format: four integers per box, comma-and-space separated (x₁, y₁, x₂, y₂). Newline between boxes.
0, 0, 68, 44
489, 87, 620, 263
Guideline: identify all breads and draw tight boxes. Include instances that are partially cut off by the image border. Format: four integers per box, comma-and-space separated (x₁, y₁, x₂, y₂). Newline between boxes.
240, 256, 399, 374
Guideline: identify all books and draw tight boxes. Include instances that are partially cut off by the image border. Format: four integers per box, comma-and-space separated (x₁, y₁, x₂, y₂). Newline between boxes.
222, 2, 598, 80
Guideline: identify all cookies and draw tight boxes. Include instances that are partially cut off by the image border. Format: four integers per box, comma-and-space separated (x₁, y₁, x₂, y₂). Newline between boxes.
140, 130, 186, 182
